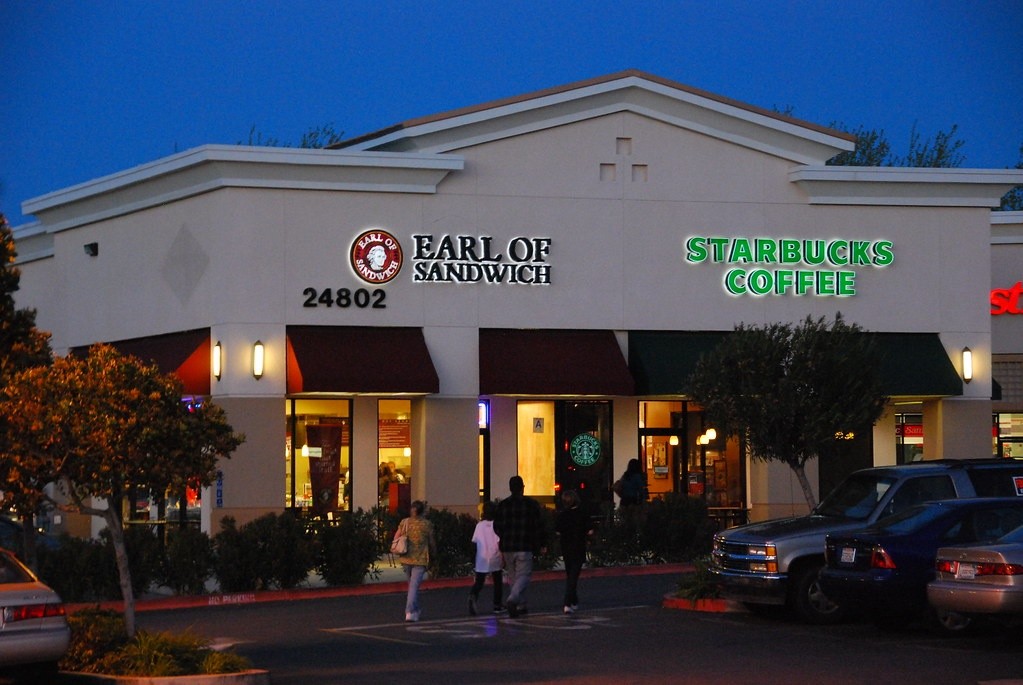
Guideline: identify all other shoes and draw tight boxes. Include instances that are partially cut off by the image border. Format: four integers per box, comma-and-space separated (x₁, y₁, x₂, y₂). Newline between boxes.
405, 612, 418, 621
571, 603, 579, 611
494, 606, 508, 614
505, 601, 522, 618
416, 609, 422, 616
563, 605, 575, 615
468, 593, 479, 616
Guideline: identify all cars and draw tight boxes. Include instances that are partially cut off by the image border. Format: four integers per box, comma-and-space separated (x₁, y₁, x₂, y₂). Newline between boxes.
0, 517, 62, 574
0, 548, 73, 681
820, 496, 1023, 630
927, 527, 1023, 636
687, 469, 714, 497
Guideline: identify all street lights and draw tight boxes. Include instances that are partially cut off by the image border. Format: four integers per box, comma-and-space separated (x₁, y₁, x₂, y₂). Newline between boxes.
694, 426, 717, 505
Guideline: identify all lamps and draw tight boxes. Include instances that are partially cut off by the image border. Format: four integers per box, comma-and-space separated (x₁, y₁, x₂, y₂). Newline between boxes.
962, 346, 972, 384
254, 340, 264, 380
213, 341, 221, 381
696, 427, 717, 446
670, 435, 679, 446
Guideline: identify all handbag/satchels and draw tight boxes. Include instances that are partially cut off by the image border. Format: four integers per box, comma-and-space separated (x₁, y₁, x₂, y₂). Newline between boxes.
612, 480, 621, 497
390, 518, 411, 555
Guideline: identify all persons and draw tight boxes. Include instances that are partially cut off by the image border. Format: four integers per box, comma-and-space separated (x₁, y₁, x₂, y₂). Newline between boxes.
706, 486, 715, 507
612, 460, 645, 523
468, 500, 508, 615
494, 477, 547, 617
339, 474, 349, 502
553, 490, 594, 613
394, 500, 436, 621
378, 464, 405, 550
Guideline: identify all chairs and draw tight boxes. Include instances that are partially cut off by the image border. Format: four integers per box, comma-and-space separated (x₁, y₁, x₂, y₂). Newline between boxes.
375, 527, 396, 568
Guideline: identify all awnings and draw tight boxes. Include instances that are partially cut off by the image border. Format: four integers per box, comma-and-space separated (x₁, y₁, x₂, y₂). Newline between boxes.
68, 328, 210, 397
479, 328, 640, 396
991, 378, 1002, 400
628, 330, 796, 396
286, 327, 439, 394
814, 332, 964, 398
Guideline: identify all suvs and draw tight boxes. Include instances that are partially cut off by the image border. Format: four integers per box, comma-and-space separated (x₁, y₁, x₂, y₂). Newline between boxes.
709, 456, 1023, 622
